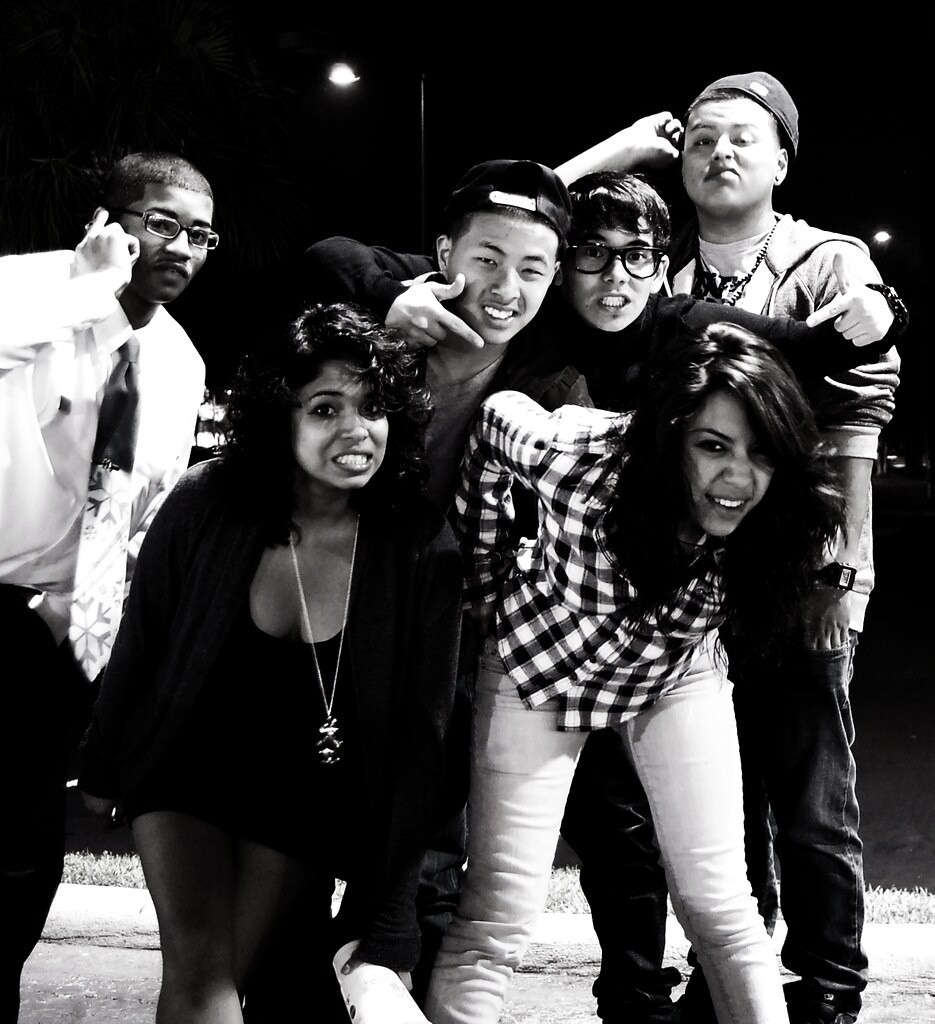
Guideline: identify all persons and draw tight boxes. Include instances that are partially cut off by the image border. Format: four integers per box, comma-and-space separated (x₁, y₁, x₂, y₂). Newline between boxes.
0, 71, 901, 1024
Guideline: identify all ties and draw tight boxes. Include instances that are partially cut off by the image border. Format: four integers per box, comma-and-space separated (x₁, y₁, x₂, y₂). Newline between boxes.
68, 336, 142, 684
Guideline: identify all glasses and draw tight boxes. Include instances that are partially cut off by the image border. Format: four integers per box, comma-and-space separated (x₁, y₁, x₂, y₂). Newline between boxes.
125, 207, 219, 250
572, 241, 664, 279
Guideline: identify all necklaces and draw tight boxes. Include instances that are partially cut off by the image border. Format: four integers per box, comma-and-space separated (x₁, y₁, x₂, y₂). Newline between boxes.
701, 222, 777, 306
450, 350, 506, 386
289, 515, 359, 763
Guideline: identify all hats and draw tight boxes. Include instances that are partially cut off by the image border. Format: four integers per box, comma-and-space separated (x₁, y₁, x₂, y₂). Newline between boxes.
699, 71, 800, 160
451, 157, 573, 250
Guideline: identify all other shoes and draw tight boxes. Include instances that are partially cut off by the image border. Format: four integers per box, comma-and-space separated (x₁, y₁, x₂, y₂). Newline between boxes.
790, 983, 862, 1024
605, 994, 684, 1024
682, 968, 724, 1024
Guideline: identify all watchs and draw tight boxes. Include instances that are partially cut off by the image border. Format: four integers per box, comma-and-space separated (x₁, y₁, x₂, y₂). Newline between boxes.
816, 562, 857, 591
865, 283, 910, 329
